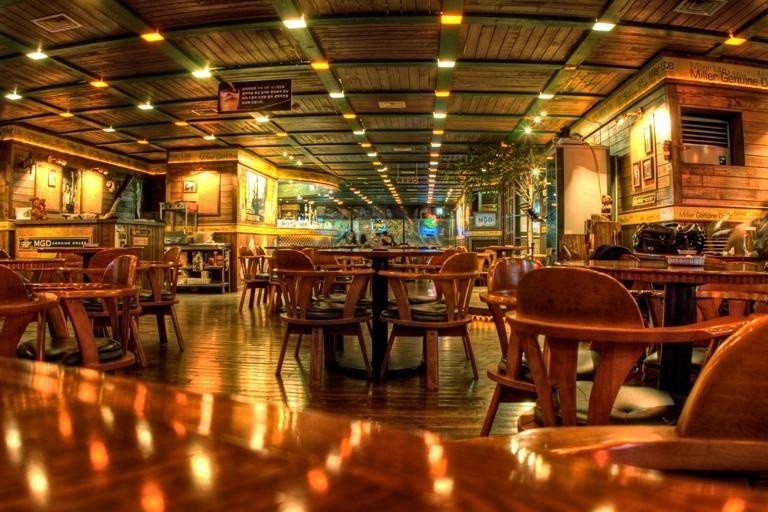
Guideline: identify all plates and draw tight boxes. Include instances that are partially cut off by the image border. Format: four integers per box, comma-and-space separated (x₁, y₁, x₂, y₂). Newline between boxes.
633, 251, 665, 261
666, 254, 707, 266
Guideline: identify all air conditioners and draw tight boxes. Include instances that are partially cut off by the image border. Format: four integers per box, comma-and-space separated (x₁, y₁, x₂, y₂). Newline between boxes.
681, 116, 732, 167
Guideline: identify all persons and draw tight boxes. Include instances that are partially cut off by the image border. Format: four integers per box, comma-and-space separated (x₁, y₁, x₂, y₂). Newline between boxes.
381, 231, 397, 246
386, 206, 396, 218
335, 230, 350, 246
348, 229, 358, 245
359, 233, 367, 244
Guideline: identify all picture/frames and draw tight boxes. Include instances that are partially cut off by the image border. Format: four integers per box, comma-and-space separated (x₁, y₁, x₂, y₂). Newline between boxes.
631, 124, 654, 187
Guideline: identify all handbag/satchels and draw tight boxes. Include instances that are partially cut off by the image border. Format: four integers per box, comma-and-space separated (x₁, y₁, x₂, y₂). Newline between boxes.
632, 222, 705, 255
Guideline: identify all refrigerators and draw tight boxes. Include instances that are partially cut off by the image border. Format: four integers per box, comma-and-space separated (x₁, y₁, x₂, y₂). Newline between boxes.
544, 142, 611, 260
